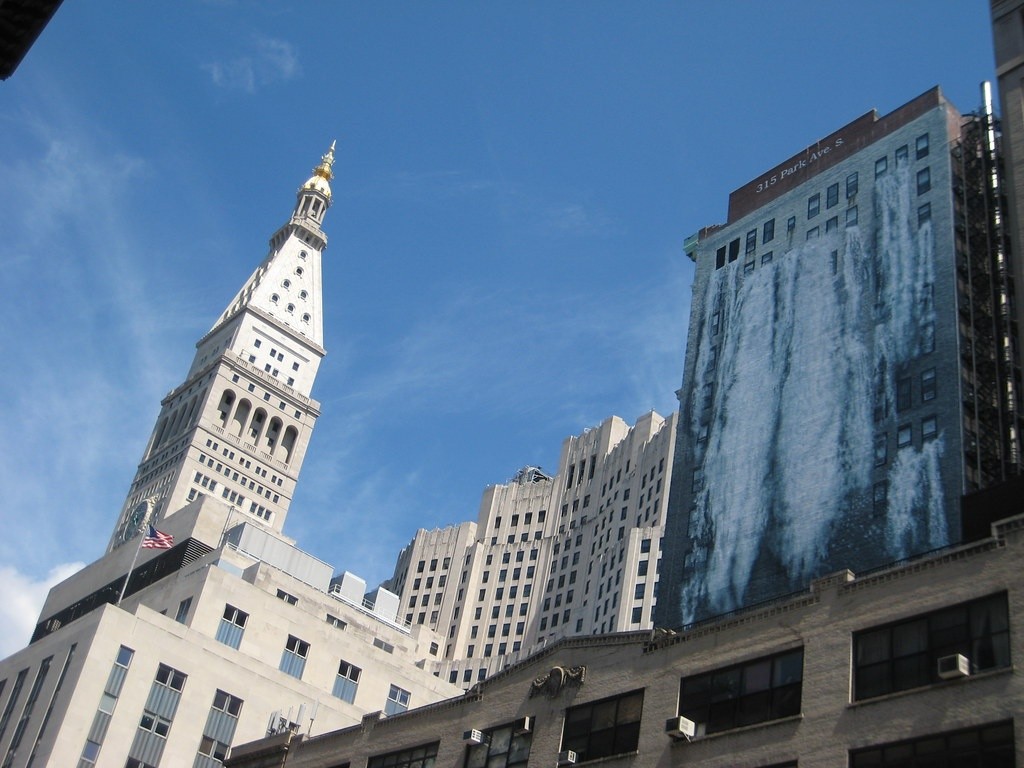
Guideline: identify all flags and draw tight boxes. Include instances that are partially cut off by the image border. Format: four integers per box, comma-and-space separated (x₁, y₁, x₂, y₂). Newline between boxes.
141, 524, 175, 550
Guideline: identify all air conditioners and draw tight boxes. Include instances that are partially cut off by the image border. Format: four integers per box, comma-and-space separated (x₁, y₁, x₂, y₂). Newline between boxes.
514, 717, 529, 733
937, 653, 969, 680
462, 728, 482, 745
665, 716, 695, 738
558, 750, 576, 765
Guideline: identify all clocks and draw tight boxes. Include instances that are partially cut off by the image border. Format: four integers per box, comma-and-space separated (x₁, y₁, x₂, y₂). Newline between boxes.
123, 499, 152, 541
